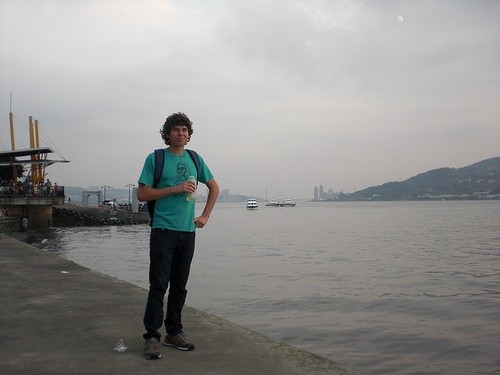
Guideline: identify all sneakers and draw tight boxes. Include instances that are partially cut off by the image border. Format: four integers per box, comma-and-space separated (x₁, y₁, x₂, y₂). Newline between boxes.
163, 331, 194, 350
143, 337, 162, 359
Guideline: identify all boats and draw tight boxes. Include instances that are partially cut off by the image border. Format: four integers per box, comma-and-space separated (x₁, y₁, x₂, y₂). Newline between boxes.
266, 202, 279, 207
246, 199, 258, 210
280, 199, 296, 207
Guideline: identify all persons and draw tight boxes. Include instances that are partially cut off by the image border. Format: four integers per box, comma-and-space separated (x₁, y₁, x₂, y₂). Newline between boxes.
0, 177, 64, 198
137, 112, 220, 360
23, 216, 28, 233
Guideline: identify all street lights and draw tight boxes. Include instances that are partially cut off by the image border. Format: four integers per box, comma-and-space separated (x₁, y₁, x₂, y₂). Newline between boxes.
125, 183, 135, 210
101, 185, 110, 201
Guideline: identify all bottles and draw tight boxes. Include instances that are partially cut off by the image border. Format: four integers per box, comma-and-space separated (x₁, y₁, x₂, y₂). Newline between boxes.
186, 176, 197, 201
